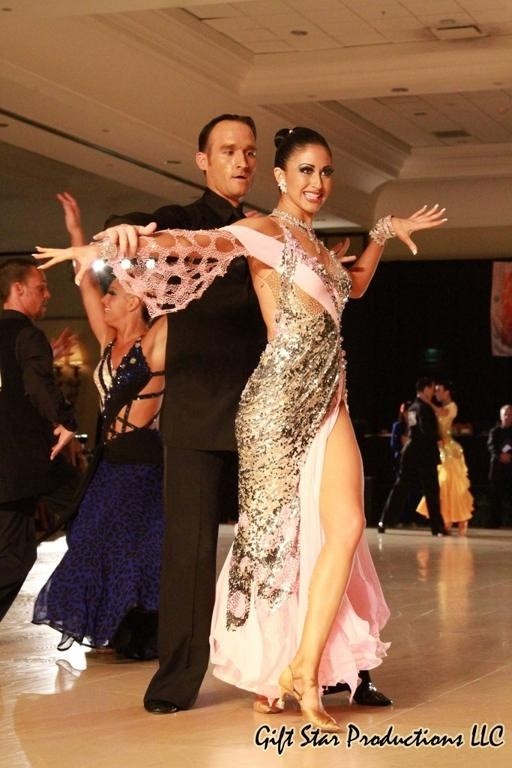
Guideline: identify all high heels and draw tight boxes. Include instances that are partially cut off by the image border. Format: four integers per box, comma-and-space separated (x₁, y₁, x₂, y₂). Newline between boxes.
460, 521, 468, 534
276, 665, 340, 733
251, 696, 279, 715
444, 522, 452, 530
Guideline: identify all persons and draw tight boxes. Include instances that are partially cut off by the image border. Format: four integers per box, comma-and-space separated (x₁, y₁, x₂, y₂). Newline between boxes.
93, 113, 267, 714
417, 380, 474, 538
32, 190, 167, 662
488, 405, 512, 529
0, 256, 89, 622
377, 378, 452, 536
391, 401, 415, 472
32, 128, 448, 733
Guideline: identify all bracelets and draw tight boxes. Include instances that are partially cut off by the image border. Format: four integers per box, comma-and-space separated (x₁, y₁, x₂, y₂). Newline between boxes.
369, 214, 394, 249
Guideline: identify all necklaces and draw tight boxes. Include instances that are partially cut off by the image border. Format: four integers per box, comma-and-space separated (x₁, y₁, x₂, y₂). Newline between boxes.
271, 206, 329, 255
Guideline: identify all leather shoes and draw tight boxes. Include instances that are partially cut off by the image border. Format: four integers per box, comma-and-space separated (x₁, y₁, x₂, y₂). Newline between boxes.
433, 526, 448, 536
377, 521, 385, 531
144, 698, 179, 714
344, 671, 392, 706
